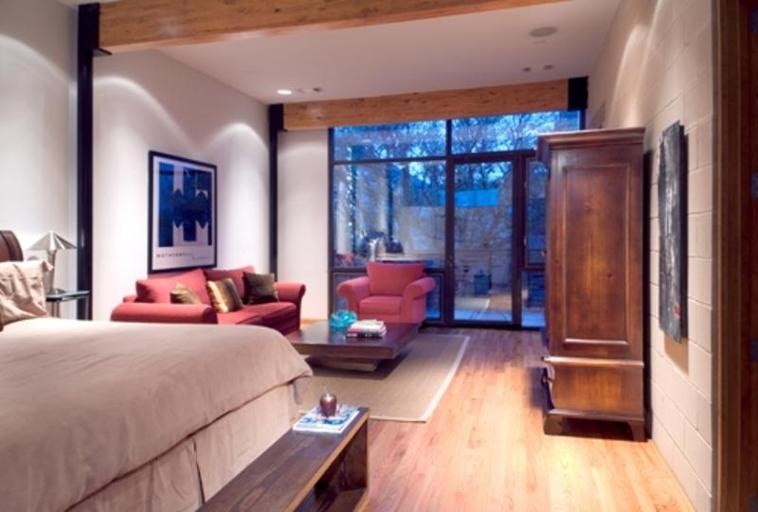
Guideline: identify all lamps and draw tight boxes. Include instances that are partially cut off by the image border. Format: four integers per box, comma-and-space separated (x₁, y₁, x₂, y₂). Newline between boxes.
27, 231, 79, 295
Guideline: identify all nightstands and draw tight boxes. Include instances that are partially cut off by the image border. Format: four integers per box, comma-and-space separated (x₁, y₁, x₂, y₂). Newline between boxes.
45, 297, 89, 322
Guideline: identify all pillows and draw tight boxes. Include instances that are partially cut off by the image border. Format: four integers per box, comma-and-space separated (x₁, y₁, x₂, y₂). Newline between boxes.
242, 270, 279, 304
206, 278, 242, 312
171, 283, 202, 304
1, 259, 52, 321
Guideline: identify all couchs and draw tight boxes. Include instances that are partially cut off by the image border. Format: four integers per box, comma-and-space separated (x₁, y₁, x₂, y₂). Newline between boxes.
110, 265, 306, 336
337, 262, 437, 323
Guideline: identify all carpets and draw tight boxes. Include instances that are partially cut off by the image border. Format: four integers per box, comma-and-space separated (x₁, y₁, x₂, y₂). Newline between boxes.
454, 295, 490, 320
300, 332, 470, 424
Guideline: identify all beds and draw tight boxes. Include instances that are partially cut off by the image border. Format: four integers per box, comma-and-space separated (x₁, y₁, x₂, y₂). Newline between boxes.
0, 231, 313, 512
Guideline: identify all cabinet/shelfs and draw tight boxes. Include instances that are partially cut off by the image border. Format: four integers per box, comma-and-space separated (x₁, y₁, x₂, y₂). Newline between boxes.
534, 127, 646, 443
196, 406, 371, 511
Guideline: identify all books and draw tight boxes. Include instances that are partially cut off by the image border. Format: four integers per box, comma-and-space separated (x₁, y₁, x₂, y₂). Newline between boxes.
292, 401, 360, 434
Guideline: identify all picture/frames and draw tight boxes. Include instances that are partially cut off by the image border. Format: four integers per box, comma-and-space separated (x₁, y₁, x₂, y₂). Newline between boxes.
147, 149, 217, 274
